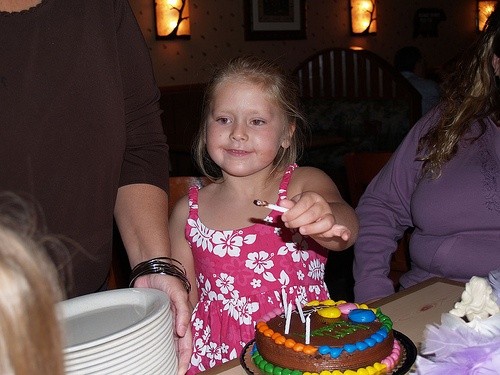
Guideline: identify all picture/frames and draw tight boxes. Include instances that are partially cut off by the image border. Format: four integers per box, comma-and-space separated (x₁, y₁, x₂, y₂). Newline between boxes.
242, 0, 307, 41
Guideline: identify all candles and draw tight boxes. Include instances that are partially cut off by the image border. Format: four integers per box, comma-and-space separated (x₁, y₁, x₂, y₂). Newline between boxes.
282, 286, 287, 318
305, 314, 311, 344
284, 300, 293, 334
295, 296, 305, 323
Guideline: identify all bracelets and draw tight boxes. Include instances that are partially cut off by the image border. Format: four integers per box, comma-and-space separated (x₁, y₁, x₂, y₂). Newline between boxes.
128, 256, 192, 294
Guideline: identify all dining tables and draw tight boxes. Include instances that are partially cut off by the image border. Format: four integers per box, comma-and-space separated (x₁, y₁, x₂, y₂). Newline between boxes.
191, 276, 468, 375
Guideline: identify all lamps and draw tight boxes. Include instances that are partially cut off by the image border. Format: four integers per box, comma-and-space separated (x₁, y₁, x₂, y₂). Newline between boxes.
476, 0, 497, 32
349, 0, 378, 35
153, 0, 192, 41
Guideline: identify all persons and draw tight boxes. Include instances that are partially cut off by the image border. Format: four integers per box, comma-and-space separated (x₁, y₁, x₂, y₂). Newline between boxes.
394, 46, 425, 80
0, 0, 193, 375
169, 56, 360, 375
354, 6, 500, 305
0, 226, 67, 375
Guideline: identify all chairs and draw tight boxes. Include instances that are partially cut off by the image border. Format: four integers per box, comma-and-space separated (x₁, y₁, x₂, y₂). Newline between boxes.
290, 46, 423, 150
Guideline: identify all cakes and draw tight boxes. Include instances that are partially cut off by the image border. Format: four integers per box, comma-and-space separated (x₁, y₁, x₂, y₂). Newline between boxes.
252, 300, 400, 375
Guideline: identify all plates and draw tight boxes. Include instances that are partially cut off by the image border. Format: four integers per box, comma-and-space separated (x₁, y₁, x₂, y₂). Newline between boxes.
54, 287, 179, 375
